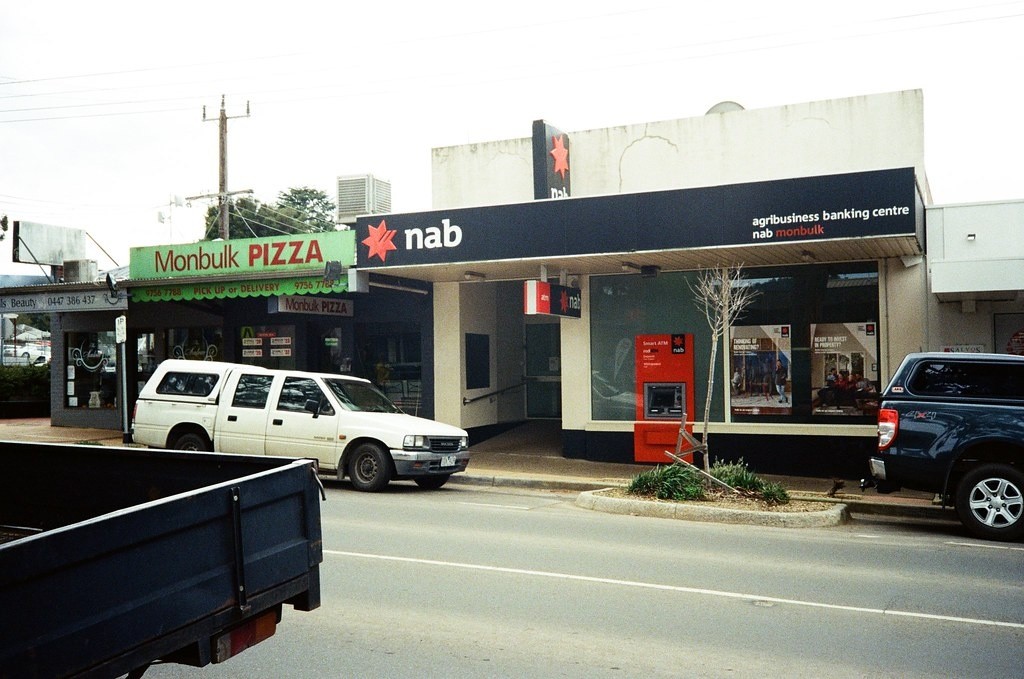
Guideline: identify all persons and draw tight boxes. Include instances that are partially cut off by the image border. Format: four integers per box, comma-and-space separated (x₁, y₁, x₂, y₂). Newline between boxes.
775, 360, 788, 403
731, 368, 742, 394
817, 368, 873, 408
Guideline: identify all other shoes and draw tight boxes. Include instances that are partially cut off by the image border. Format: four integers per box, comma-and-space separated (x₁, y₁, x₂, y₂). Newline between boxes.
778, 400, 782, 403
785, 397, 788, 403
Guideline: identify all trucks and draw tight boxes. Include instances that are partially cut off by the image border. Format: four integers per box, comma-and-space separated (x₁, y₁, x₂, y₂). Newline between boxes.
0, 438, 326, 679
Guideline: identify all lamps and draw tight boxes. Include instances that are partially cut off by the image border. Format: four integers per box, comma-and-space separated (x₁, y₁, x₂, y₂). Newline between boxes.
621, 261, 642, 273
463, 270, 486, 282
324, 260, 348, 287
801, 249, 817, 264
105, 272, 134, 298
641, 265, 660, 278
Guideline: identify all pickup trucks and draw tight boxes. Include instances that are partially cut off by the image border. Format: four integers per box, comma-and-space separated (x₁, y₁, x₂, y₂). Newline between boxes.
122, 358, 472, 495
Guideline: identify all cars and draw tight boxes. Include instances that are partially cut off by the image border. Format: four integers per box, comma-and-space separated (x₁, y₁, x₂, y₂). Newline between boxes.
869, 350, 1024, 541
4, 343, 51, 363
